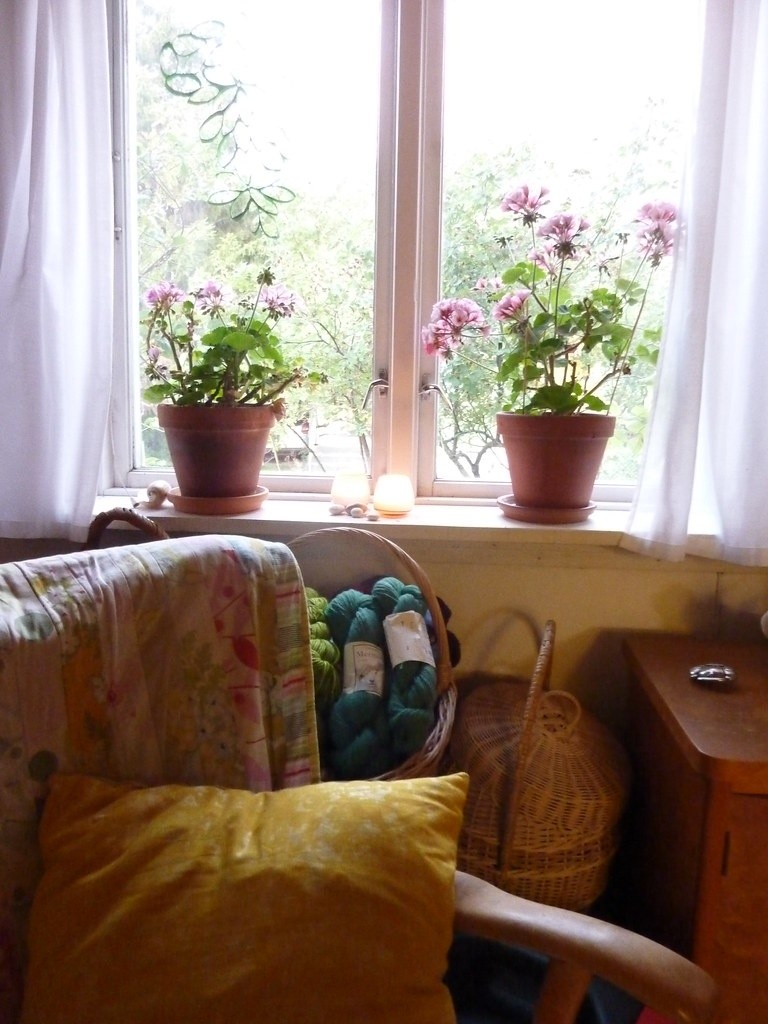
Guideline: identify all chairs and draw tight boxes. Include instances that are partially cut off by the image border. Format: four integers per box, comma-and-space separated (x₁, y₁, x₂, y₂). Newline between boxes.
0, 534, 730, 1024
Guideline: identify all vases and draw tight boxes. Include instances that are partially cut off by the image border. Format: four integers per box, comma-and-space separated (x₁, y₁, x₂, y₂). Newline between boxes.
157, 403, 275, 515
496, 411, 616, 524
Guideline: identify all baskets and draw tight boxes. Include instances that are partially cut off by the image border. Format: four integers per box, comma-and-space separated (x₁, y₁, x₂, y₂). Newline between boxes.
443, 618, 630, 905
281, 528, 457, 789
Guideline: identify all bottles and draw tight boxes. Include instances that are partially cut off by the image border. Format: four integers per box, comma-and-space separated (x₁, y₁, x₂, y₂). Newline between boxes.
331, 470, 370, 510
373, 474, 416, 516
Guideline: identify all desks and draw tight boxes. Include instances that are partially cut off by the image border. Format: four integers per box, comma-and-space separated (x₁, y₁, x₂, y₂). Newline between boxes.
624, 637, 768, 1024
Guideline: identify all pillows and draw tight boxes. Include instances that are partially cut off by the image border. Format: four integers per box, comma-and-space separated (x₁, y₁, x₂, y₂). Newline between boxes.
20, 770, 470, 1024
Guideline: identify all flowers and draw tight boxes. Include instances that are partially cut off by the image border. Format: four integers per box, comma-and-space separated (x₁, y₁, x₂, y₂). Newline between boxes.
420, 181, 682, 414
139, 266, 331, 406
